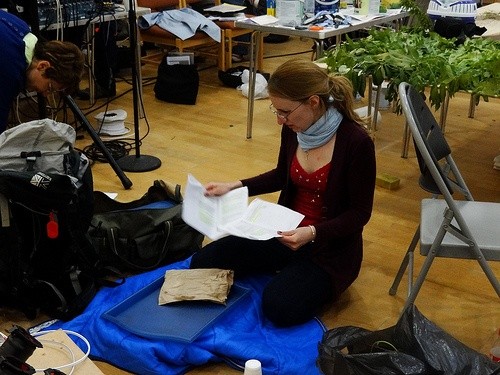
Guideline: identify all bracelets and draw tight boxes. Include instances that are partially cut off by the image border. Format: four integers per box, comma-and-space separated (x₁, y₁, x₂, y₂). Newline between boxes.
309, 225, 316, 242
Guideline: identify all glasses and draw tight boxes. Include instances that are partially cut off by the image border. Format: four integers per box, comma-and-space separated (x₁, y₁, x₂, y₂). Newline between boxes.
268, 102, 305, 121
45, 79, 58, 94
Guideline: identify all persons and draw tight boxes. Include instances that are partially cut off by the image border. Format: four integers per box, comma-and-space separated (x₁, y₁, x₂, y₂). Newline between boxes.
0, 9, 85, 136
190, 59, 376, 329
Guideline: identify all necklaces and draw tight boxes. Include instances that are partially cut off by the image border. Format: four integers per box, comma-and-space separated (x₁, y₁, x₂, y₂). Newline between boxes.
305, 151, 309, 160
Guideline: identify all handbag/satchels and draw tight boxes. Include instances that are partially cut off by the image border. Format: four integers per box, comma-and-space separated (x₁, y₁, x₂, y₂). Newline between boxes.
218, 65, 272, 89
153, 53, 200, 105
76, 178, 205, 273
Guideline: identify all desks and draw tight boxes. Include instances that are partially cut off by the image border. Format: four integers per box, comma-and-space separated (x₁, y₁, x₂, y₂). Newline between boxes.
234, 9, 411, 139
315, 2, 500, 158
29, 4, 151, 105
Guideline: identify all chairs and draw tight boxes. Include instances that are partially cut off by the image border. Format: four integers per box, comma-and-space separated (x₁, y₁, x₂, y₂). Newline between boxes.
389, 82, 500, 313
135, 0, 257, 71
